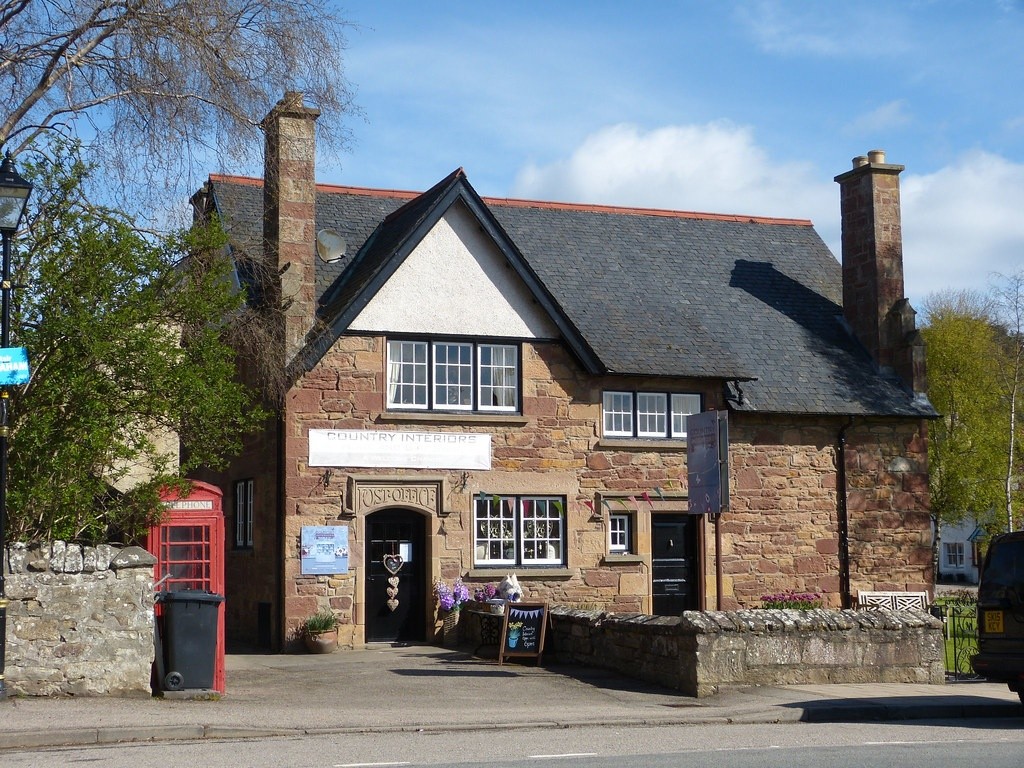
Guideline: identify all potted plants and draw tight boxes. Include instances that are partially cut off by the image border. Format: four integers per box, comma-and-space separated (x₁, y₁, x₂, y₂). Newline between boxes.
301, 612, 338, 653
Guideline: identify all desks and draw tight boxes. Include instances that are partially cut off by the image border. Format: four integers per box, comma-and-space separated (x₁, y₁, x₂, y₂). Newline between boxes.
469, 610, 505, 655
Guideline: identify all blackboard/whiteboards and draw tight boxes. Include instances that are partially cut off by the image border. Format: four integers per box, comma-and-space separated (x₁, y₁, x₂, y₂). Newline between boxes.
499, 602, 553, 656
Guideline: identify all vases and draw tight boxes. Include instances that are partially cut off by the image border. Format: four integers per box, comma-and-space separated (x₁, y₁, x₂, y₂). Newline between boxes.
483, 602, 495, 612
490, 604, 504, 614
443, 612, 461, 647
475, 602, 483, 611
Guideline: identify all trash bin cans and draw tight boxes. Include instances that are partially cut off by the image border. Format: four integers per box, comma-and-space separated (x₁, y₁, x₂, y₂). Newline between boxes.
154, 588, 226, 691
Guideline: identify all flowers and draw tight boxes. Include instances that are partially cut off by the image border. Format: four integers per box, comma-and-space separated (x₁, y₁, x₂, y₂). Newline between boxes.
433, 577, 469, 613
474, 592, 484, 602
504, 588, 520, 603
484, 584, 496, 602
